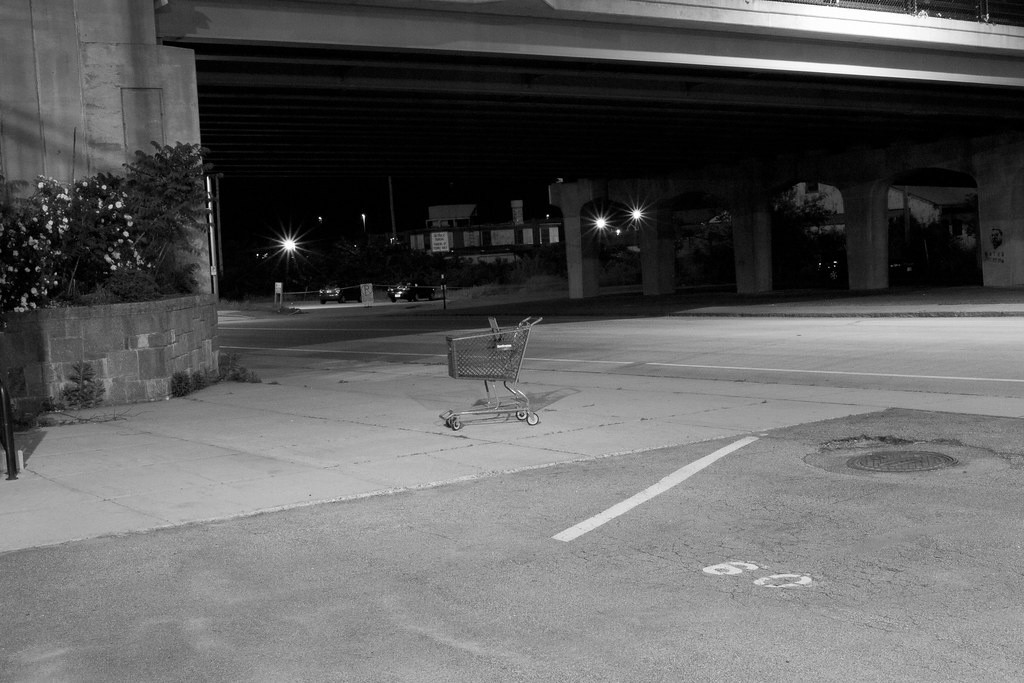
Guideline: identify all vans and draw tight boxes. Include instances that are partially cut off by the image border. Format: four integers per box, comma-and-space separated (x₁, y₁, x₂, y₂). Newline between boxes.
319, 280, 362, 304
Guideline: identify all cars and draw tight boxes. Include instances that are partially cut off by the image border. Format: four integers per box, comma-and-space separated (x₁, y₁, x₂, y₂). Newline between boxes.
387, 279, 435, 303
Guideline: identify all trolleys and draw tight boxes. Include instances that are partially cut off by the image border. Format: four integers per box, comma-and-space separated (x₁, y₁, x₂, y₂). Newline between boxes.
439, 315, 543, 430
